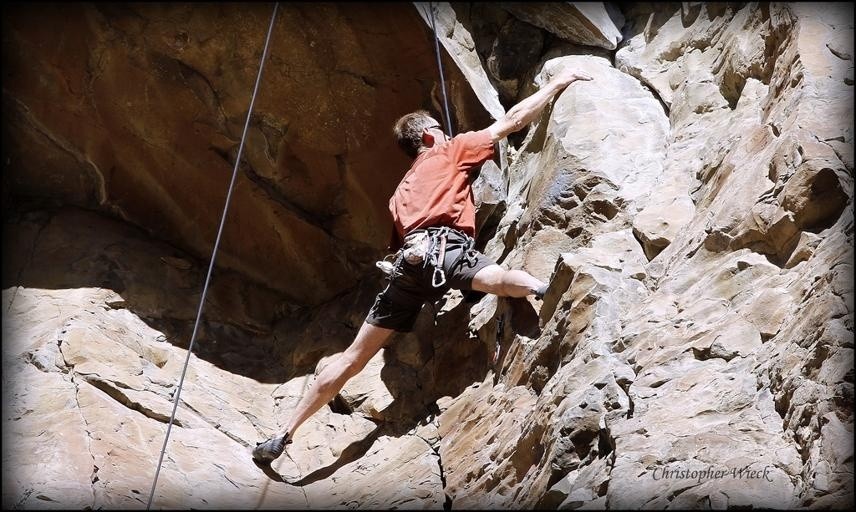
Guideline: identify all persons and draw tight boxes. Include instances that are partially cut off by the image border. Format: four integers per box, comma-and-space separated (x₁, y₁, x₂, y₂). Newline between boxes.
251, 71, 594, 462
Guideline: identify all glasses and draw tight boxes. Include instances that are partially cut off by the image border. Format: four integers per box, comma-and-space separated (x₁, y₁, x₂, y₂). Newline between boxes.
422, 124, 445, 143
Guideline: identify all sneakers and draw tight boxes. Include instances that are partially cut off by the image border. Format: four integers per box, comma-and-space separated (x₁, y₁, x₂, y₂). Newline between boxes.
251, 432, 292, 462
